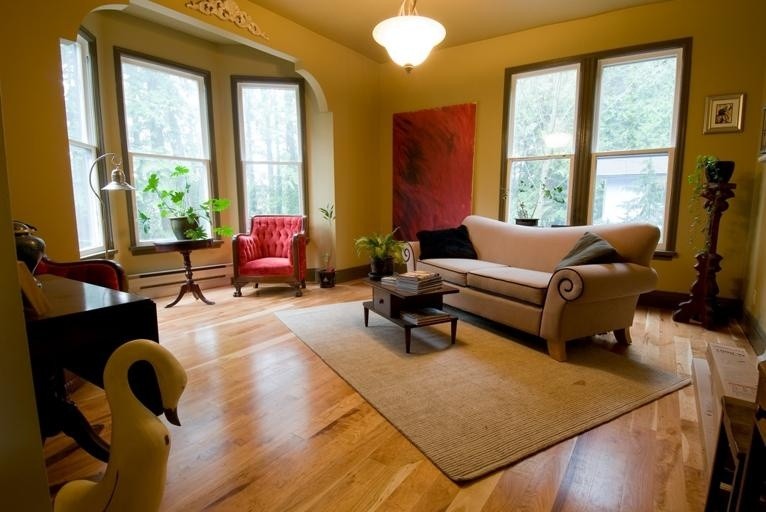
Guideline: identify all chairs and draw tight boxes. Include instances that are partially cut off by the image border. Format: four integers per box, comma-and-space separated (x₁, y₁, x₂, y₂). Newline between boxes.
34, 257, 129, 293
230, 213, 311, 298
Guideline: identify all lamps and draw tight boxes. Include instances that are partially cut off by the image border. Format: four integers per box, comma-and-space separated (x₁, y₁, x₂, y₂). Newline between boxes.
372, 1, 448, 78
87, 151, 137, 261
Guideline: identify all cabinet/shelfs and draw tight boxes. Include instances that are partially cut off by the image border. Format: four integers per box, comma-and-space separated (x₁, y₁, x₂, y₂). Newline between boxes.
704, 360, 766, 511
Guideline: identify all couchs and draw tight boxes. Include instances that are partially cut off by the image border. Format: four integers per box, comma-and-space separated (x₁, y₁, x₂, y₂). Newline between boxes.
401, 214, 664, 363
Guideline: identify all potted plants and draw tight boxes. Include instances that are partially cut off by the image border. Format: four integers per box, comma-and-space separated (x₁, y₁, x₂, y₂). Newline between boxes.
136, 163, 235, 241
495, 172, 569, 226
351, 224, 408, 281
683, 149, 737, 256
315, 201, 340, 289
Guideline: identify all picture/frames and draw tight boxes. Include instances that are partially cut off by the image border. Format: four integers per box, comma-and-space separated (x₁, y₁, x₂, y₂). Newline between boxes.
757, 106, 766, 164
701, 92, 747, 136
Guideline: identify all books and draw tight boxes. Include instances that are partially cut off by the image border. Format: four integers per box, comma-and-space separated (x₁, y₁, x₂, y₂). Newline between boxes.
381, 269, 452, 326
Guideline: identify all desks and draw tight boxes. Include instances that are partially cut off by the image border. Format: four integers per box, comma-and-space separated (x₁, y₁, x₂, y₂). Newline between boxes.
361, 280, 461, 354
24, 272, 160, 463
152, 237, 222, 309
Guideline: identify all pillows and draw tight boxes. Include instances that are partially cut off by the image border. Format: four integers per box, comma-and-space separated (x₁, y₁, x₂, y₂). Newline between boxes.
553, 230, 618, 271
415, 223, 477, 262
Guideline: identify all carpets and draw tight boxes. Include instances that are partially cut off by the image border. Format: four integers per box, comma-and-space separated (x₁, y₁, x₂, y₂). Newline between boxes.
272, 298, 695, 489
